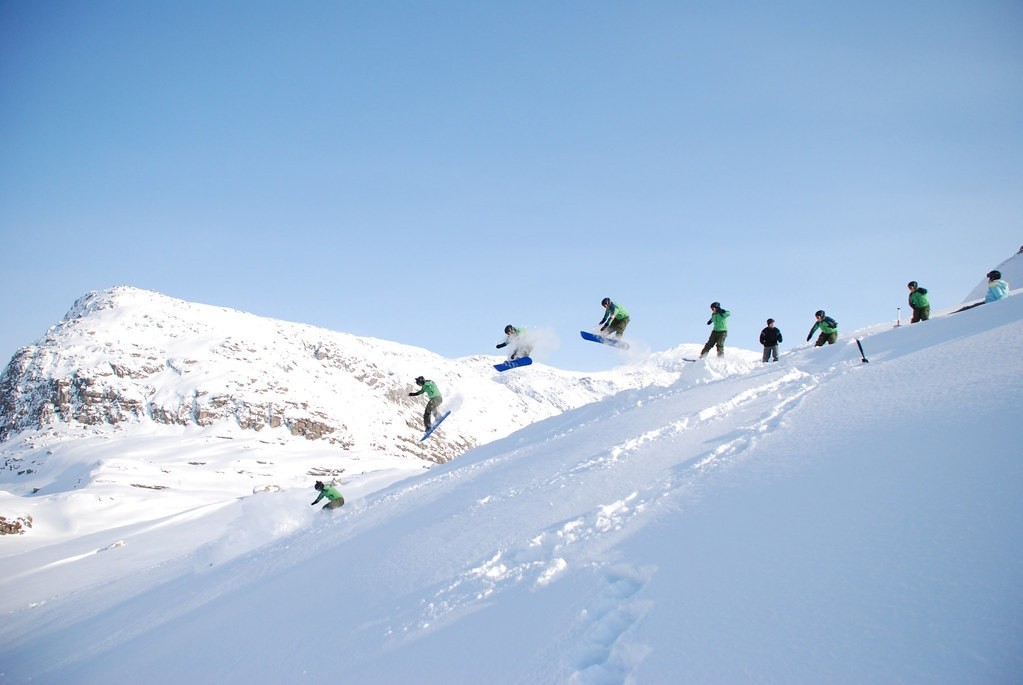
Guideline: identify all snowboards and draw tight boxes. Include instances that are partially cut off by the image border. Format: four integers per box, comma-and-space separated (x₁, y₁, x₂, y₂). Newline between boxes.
579, 329, 631, 349
493, 356, 532, 372
418, 410, 452, 442
682, 357, 698, 362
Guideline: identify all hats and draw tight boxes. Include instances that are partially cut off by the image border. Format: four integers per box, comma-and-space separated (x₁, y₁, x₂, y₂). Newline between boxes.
505, 325, 513, 334
416, 376, 425, 384
602, 298, 611, 305
816, 310, 825, 317
987, 270, 1001, 281
767, 318, 775, 325
314, 482, 324, 491
711, 302, 720, 308
908, 281, 917, 287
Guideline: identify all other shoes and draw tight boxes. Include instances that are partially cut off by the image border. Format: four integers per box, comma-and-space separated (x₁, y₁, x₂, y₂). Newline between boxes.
435, 415, 442, 422
425, 427, 431, 432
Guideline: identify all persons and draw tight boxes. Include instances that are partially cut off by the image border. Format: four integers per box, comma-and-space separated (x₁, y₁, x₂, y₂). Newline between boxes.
496, 325, 532, 359
807, 310, 838, 346
984, 271, 1008, 303
409, 376, 442, 432
760, 319, 782, 363
599, 298, 630, 339
311, 481, 344, 510
908, 281, 930, 323
698, 302, 731, 358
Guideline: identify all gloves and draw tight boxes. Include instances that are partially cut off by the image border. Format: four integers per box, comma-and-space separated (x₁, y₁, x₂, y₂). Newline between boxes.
827, 323, 835, 328
311, 500, 318, 505
496, 343, 506, 349
806, 335, 812, 342
599, 318, 605, 325
601, 324, 610, 331
718, 309, 725, 313
708, 320, 712, 325
512, 354, 516, 360
